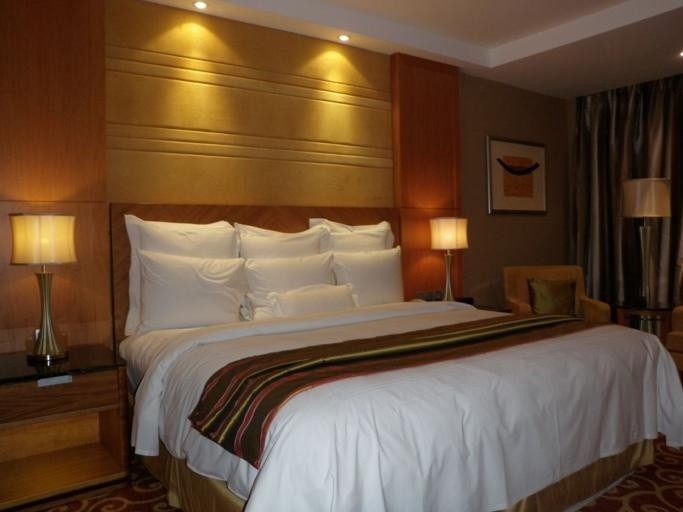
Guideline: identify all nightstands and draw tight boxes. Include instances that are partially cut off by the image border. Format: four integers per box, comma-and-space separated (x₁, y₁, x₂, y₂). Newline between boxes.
460, 299, 510, 315
0, 340, 132, 512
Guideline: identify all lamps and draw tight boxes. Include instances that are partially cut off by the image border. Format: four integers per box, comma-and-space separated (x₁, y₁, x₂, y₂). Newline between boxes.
429, 216, 468, 304
7, 210, 81, 361
620, 176, 670, 312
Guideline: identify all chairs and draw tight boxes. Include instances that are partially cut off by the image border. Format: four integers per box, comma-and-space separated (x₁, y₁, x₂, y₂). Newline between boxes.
499, 262, 610, 322
661, 304, 682, 375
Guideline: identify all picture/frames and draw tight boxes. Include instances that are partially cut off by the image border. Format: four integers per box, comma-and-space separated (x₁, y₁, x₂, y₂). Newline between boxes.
484, 133, 549, 215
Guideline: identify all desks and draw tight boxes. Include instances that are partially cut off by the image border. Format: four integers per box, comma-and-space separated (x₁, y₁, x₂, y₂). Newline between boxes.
610, 301, 671, 346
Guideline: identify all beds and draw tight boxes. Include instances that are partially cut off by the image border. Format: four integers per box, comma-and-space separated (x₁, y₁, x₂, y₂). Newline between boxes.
104, 196, 682, 512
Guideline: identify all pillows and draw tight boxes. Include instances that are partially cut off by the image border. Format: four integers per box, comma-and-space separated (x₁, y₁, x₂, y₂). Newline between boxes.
528, 276, 577, 317
121, 211, 405, 341
